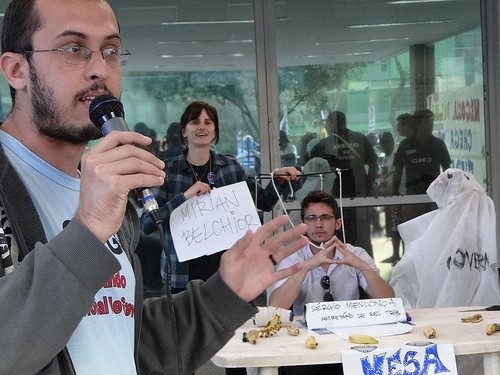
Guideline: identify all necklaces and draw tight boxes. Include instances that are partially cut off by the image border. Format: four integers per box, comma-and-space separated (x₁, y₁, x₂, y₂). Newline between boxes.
190, 155, 211, 188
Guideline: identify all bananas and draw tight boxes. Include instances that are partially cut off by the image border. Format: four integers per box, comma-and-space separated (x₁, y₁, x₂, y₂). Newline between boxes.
486, 322, 500, 336
247, 329, 263, 344
305, 336, 318, 348
349, 334, 378, 344
287, 324, 300, 335
461, 313, 483, 322
423, 325, 436, 339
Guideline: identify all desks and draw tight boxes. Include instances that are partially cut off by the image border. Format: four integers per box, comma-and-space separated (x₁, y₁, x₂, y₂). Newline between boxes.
210, 306, 500, 375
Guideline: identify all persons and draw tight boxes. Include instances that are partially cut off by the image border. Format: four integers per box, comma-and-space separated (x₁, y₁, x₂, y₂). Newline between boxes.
142, 101, 303, 292
267, 189, 396, 317
0, 0, 309, 375
133, 121, 186, 207
237, 110, 453, 265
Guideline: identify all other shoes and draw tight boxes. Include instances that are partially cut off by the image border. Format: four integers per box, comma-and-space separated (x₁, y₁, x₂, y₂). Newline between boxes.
380, 256, 399, 263
391, 259, 401, 266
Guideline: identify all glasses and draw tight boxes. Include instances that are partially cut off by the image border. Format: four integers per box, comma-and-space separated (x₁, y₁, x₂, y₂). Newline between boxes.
302, 215, 336, 223
320, 276, 334, 302
24, 46, 130, 68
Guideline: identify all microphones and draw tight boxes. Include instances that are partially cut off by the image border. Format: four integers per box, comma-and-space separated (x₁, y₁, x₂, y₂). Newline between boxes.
89, 94, 163, 225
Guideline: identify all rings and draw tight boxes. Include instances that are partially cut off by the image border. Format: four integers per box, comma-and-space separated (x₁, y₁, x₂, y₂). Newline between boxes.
269, 254, 277, 265
345, 248, 348, 250
